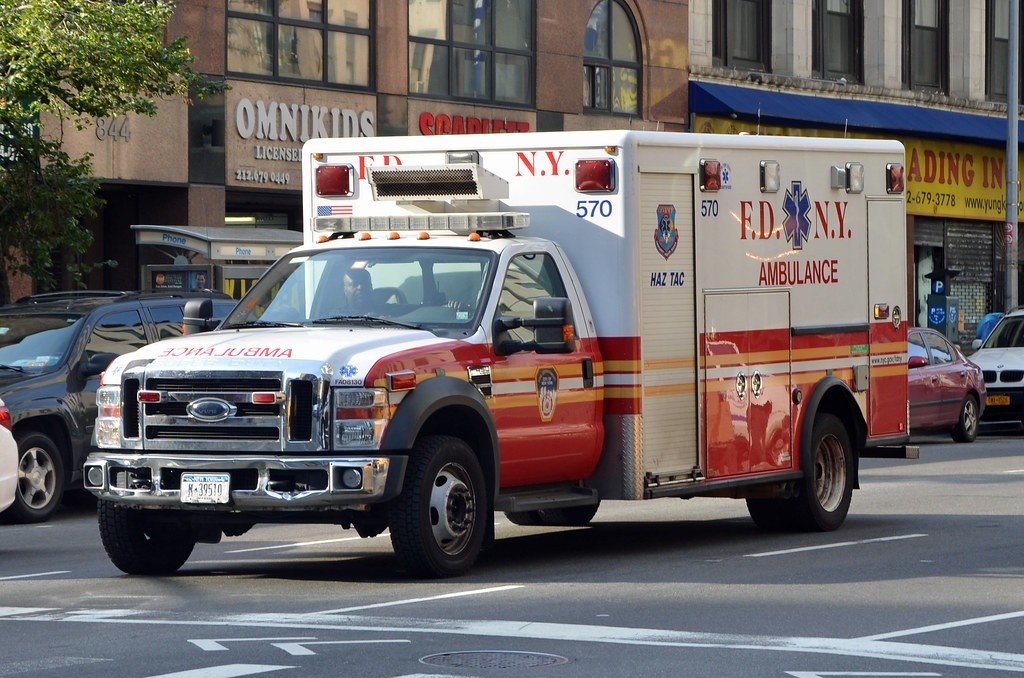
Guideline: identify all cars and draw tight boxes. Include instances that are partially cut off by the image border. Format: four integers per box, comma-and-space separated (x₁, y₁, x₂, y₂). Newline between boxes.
909, 326, 987, 443
1, 401, 20, 511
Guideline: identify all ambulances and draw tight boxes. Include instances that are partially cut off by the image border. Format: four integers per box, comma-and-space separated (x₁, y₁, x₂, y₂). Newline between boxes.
83, 130, 919, 577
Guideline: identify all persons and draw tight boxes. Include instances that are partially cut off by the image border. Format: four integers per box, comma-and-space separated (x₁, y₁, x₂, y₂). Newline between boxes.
326, 269, 376, 320
443, 269, 499, 312
190, 273, 209, 290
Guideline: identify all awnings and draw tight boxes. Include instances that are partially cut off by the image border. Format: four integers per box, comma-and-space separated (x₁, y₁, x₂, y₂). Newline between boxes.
686, 78, 1024, 147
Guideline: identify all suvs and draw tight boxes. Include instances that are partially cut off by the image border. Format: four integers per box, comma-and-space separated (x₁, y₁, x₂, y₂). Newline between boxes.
0, 290, 258, 522
968, 305, 1024, 435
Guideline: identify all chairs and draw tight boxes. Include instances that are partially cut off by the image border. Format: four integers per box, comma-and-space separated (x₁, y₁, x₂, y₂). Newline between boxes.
374, 287, 407, 304
